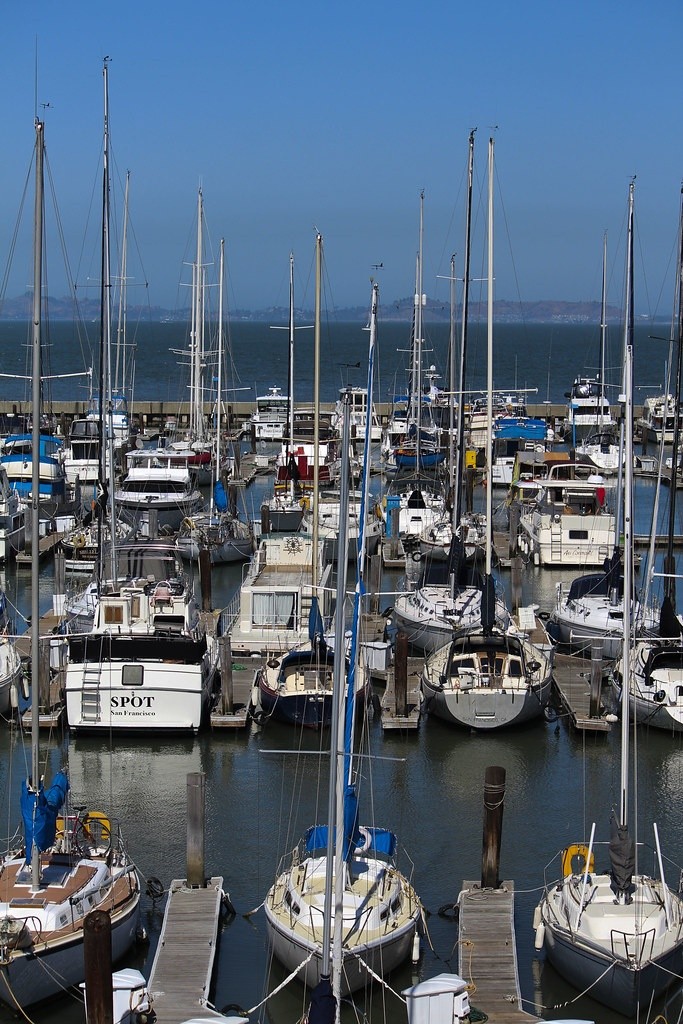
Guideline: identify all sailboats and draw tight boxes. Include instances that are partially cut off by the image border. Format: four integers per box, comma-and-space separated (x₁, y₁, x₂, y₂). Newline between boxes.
0, 43, 683, 1024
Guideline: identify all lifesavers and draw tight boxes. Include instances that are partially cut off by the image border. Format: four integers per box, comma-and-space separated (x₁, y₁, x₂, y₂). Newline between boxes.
55, 816, 65, 841
561, 843, 595, 878
83, 811, 109, 840
146, 877, 164, 898
224, 898, 237, 915
352, 825, 372, 854
298, 498, 310, 510
